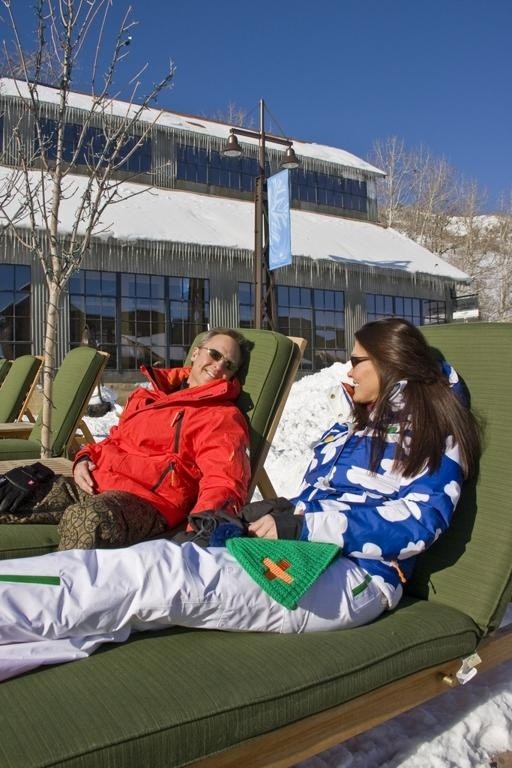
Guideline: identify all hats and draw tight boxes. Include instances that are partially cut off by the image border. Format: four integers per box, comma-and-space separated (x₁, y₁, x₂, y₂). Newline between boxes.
208, 522, 342, 610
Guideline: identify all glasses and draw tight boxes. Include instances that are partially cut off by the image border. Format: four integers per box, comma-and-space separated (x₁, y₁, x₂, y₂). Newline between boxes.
199, 346, 239, 370
351, 357, 369, 367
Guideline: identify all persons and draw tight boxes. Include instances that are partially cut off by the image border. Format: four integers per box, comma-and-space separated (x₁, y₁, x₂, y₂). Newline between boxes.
1, 318, 483, 682
0, 326, 253, 552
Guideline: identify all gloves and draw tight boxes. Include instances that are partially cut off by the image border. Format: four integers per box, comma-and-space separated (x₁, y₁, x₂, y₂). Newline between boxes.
0, 461, 55, 514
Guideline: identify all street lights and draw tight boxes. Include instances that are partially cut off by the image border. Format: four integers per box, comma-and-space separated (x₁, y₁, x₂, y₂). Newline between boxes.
221, 99, 300, 334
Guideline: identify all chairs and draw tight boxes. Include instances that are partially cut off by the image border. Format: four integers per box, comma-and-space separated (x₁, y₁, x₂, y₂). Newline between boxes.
1, 320, 512, 768
